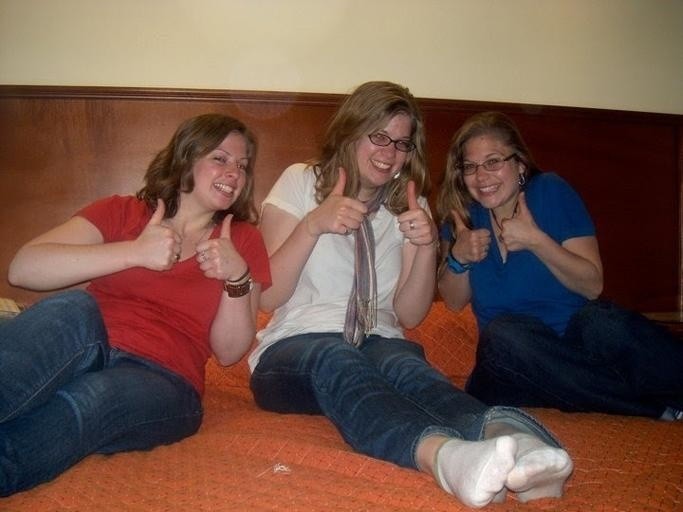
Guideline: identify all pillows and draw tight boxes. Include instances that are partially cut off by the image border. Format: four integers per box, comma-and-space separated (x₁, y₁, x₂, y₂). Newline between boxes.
193, 303, 275, 399
405, 299, 481, 378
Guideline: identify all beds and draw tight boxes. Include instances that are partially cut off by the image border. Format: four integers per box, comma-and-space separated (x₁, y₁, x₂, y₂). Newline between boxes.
0, 299, 683, 512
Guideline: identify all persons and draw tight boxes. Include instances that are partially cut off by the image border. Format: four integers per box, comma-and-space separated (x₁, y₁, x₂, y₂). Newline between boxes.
242, 78, 576, 511
0, 110, 275, 506
430, 110, 682, 424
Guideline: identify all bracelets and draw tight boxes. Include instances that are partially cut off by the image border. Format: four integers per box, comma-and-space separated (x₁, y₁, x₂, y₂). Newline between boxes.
222, 266, 253, 298
445, 249, 471, 275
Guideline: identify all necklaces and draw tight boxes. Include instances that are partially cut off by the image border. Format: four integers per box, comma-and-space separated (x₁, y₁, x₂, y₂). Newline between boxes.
489, 201, 519, 242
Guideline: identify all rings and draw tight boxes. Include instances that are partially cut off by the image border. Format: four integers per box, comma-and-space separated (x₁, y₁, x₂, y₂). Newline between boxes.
408, 220, 415, 230
174, 253, 179, 260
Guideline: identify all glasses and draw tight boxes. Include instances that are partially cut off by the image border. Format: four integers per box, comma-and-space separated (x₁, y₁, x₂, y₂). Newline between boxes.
460, 155, 515, 176
366, 132, 416, 153
